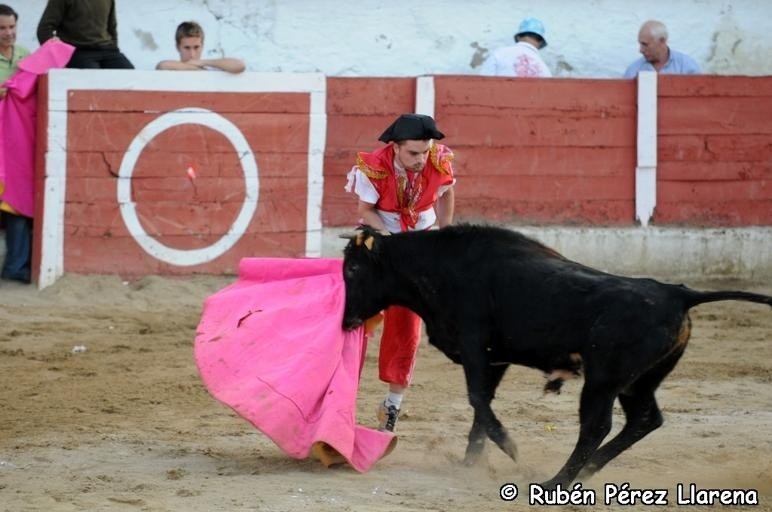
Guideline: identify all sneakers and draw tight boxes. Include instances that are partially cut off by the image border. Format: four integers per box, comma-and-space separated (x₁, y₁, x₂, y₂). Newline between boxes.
375, 400, 401, 433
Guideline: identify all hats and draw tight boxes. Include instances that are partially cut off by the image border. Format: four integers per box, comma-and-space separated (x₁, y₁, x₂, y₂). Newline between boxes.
513, 17, 548, 50
378, 114, 445, 144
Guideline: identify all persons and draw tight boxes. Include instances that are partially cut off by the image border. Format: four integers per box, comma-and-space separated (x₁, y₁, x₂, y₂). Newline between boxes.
344, 113, 458, 433
623, 20, 700, 79
38, 0, 137, 69
156, 22, 246, 75
475, 16, 564, 79
1, 3, 35, 283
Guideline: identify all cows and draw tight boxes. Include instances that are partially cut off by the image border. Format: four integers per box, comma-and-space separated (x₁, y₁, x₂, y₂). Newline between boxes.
337, 223, 771, 496
